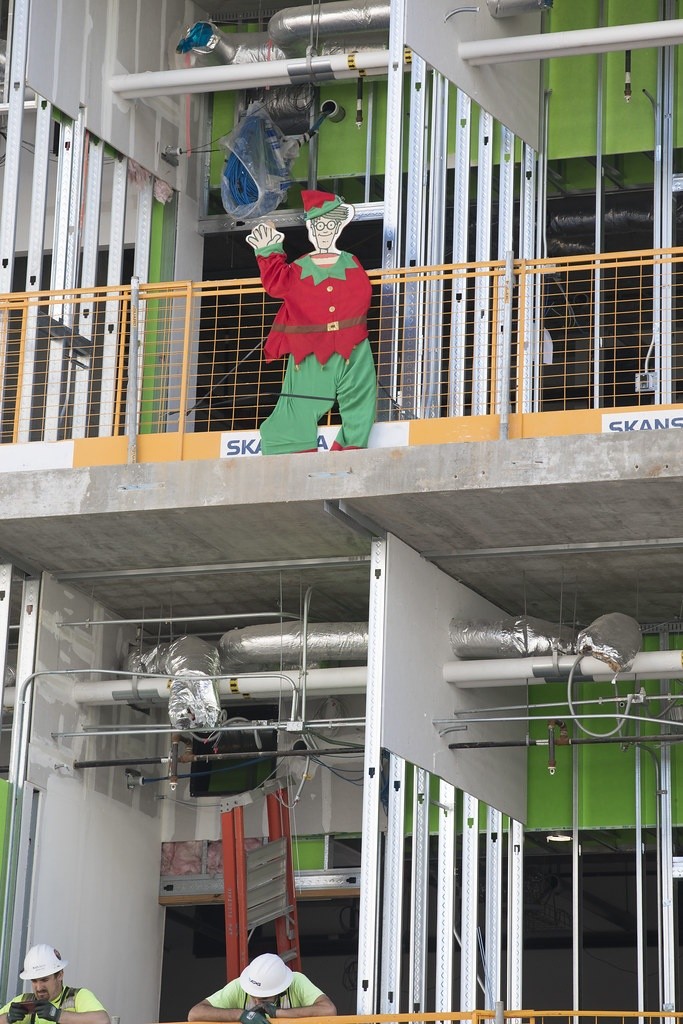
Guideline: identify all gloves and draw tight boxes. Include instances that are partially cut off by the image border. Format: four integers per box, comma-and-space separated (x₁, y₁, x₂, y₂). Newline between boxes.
31, 999, 60, 1023
6, 1000, 26, 1022
239, 1001, 275, 1023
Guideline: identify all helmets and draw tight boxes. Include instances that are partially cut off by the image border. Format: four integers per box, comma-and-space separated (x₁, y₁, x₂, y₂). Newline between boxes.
19, 943, 69, 980
239, 952, 292, 997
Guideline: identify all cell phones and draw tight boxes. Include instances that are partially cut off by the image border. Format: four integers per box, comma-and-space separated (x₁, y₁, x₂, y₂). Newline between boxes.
16, 1001, 36, 1014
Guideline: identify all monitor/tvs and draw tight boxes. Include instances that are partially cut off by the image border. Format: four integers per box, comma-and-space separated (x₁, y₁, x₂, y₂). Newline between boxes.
189, 703, 287, 797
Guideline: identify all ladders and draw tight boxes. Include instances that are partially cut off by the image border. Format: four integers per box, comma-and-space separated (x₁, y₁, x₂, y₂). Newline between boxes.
219, 774, 301, 985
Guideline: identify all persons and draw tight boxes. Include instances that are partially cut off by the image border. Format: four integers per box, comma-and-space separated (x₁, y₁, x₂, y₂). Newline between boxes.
0, 946, 110, 1023
187, 953, 337, 1024
